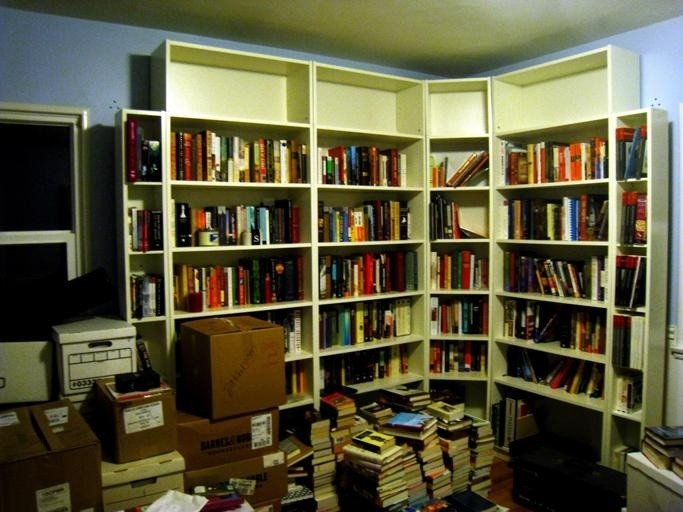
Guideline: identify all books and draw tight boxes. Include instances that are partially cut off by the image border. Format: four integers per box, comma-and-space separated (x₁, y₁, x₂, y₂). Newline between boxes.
429, 340, 488, 376
611, 313, 645, 371
616, 375, 643, 416
640, 424, 683, 479
126, 118, 162, 184
489, 396, 541, 449
320, 248, 420, 300
505, 344, 604, 400
619, 191, 647, 243
431, 297, 487, 338
317, 144, 408, 187
499, 136, 607, 186
502, 193, 608, 243
285, 362, 306, 395
130, 269, 166, 320
613, 125, 648, 182
319, 344, 411, 397
316, 198, 413, 242
170, 197, 301, 248
169, 129, 309, 183
174, 254, 306, 314
502, 298, 606, 354
430, 150, 489, 187
429, 192, 486, 240
280, 384, 510, 512
428, 252, 489, 290
126, 206, 163, 251
502, 250, 611, 303
612, 443, 635, 472
253, 308, 306, 355
614, 254, 646, 310
319, 298, 413, 349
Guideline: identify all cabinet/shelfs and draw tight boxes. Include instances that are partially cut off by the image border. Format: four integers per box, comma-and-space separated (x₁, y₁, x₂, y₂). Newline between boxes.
112, 37, 427, 414
426, 43, 669, 475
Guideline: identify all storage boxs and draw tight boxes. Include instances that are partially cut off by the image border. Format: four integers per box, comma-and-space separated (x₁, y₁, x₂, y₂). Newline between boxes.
184, 449, 289, 504
0, 398, 102, 511
180, 314, 287, 421
97, 374, 177, 463
177, 408, 281, 471
100, 451, 185, 511
50, 315, 136, 397
